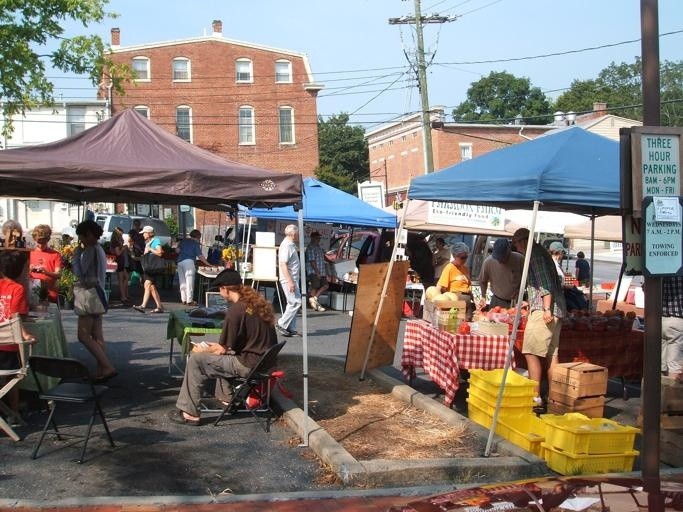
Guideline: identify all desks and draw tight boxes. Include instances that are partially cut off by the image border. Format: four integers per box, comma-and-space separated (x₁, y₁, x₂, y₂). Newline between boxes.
198, 268, 252, 303
19, 302, 68, 394
401, 320, 516, 410
509, 328, 644, 401
166, 308, 222, 379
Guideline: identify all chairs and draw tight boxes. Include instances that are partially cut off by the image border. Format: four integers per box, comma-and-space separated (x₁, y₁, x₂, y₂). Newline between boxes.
214, 340, 286, 432
1, 338, 37, 440
28, 355, 115, 463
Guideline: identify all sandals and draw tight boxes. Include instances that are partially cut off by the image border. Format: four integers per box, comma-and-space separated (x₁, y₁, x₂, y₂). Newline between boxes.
169, 410, 200, 425
201, 399, 232, 412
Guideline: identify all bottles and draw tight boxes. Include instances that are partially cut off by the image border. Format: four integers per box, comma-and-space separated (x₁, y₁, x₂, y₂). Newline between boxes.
15, 237, 27, 248
448, 308, 457, 332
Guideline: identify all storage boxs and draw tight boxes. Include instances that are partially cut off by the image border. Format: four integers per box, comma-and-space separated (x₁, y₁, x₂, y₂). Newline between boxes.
402, 299, 420, 317
332, 291, 356, 310
547, 363, 609, 417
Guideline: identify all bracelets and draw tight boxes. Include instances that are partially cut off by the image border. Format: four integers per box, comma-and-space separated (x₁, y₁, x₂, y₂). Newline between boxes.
543, 308, 552, 312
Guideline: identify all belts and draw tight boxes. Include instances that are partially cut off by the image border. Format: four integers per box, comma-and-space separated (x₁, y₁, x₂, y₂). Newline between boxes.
495, 295, 511, 302
462, 292, 470, 295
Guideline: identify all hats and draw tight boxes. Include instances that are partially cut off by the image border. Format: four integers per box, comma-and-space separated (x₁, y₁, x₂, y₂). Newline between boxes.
139, 226, 153, 233
550, 242, 567, 253
493, 239, 508, 259
211, 270, 240, 286
311, 232, 322, 236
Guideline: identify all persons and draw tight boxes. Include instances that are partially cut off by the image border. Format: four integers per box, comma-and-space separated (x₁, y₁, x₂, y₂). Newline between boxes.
431, 238, 450, 286
61, 220, 119, 385
512, 228, 567, 414
436, 242, 474, 304
478, 238, 525, 310
305, 232, 333, 312
547, 241, 567, 286
0, 250, 39, 428
168, 269, 277, 426
0, 219, 212, 313
575, 252, 591, 285
661, 276, 683, 384
274, 224, 302, 337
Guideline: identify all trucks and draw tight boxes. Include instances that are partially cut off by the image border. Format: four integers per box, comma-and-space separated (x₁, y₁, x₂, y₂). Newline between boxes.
213, 222, 333, 253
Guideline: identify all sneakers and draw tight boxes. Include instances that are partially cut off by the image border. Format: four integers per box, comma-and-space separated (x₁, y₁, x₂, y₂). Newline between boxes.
275, 296, 325, 337
121, 297, 197, 313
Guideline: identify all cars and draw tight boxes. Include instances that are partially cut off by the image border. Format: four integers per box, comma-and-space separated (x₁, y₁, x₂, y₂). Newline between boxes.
324, 230, 379, 264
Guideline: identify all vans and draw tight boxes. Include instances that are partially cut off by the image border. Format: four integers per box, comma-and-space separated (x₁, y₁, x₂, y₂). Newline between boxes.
61, 211, 172, 248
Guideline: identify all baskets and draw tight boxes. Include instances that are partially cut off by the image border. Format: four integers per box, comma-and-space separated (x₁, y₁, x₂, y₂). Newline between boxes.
466, 368, 641, 476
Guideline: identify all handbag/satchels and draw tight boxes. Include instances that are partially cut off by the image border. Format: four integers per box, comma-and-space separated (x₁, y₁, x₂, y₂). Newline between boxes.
140, 255, 165, 274
564, 286, 586, 310
73, 284, 108, 316
245, 377, 276, 410
